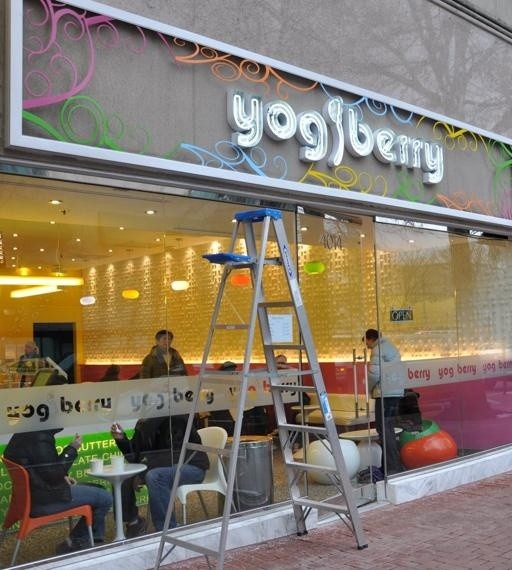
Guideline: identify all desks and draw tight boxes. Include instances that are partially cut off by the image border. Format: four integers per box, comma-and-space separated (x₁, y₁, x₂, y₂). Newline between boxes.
86, 464, 147, 542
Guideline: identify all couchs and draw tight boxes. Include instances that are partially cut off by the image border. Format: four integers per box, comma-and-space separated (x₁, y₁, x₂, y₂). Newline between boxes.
295, 393, 376, 432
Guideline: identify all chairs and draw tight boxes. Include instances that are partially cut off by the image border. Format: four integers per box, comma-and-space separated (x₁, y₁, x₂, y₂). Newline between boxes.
0, 457, 95, 567
177, 427, 237, 525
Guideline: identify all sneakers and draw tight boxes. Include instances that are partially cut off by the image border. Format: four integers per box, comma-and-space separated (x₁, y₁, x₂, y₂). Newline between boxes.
126, 517, 147, 536
57, 541, 70, 553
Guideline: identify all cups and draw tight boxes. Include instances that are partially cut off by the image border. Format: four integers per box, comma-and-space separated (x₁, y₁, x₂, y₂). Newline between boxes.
109, 455, 125, 474
89, 459, 104, 474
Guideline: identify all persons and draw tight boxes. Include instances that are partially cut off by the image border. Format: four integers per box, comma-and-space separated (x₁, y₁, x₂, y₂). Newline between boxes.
266, 354, 311, 438
110, 413, 210, 539
6, 429, 113, 555
363, 330, 406, 479
18, 343, 42, 387
141, 330, 186, 378
207, 362, 244, 430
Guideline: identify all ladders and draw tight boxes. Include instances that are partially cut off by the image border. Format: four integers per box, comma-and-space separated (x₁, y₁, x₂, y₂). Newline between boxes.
154, 208, 369, 569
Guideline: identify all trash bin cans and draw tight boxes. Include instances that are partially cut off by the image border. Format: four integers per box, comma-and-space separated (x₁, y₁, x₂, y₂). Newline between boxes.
218, 436, 273, 516
